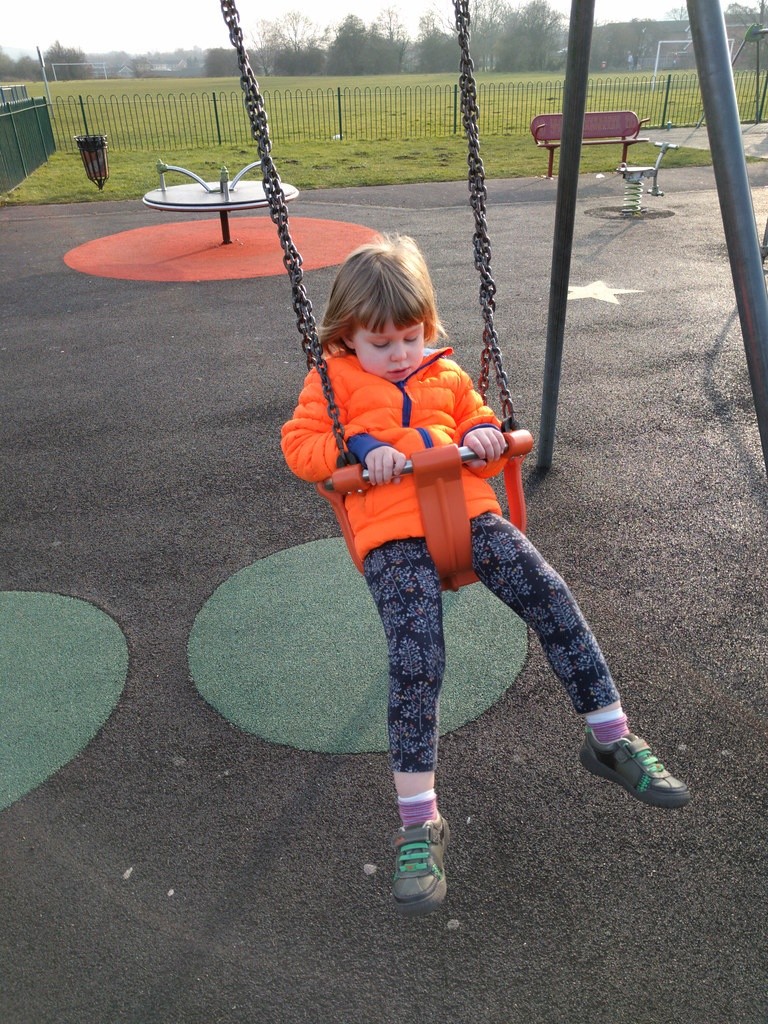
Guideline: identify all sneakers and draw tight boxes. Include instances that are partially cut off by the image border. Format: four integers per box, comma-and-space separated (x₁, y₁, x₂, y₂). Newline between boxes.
577, 730, 691, 809
391, 814, 451, 914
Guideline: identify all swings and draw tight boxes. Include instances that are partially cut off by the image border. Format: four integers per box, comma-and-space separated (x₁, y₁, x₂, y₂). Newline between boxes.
217, 1, 539, 595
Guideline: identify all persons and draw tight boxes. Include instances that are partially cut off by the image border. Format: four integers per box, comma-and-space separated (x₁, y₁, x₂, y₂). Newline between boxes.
279, 237, 690, 916
